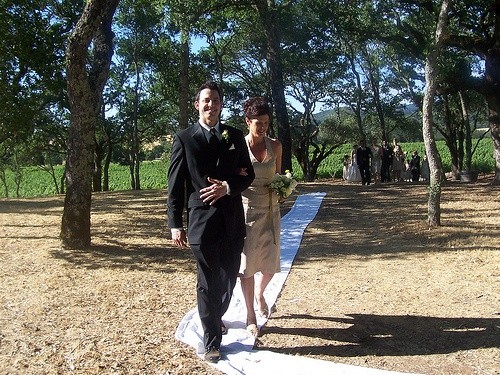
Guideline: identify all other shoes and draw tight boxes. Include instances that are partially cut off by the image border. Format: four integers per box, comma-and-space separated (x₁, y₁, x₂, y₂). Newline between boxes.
367, 183, 369, 185
362, 183, 365, 186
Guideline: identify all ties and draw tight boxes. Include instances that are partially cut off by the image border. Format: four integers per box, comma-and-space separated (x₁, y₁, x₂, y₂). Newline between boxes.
362, 148, 364, 151
206, 129, 222, 187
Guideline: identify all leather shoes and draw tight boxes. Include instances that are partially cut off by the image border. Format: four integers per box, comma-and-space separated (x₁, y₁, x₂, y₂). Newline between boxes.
204, 347, 220, 362
221, 320, 227, 335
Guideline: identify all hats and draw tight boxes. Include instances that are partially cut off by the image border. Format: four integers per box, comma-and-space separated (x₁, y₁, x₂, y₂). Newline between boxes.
344, 155, 351, 157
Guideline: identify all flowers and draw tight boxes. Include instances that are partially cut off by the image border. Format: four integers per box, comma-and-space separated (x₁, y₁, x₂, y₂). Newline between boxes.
220, 130, 230, 142
265, 169, 297, 203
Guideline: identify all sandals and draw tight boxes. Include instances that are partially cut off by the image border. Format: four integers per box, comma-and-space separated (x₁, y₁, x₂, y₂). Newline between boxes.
245, 312, 258, 337
254, 290, 269, 319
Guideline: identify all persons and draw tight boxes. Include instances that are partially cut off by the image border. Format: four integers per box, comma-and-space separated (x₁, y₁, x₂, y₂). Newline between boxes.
342, 138, 431, 187
166, 82, 255, 364
237, 98, 286, 336
